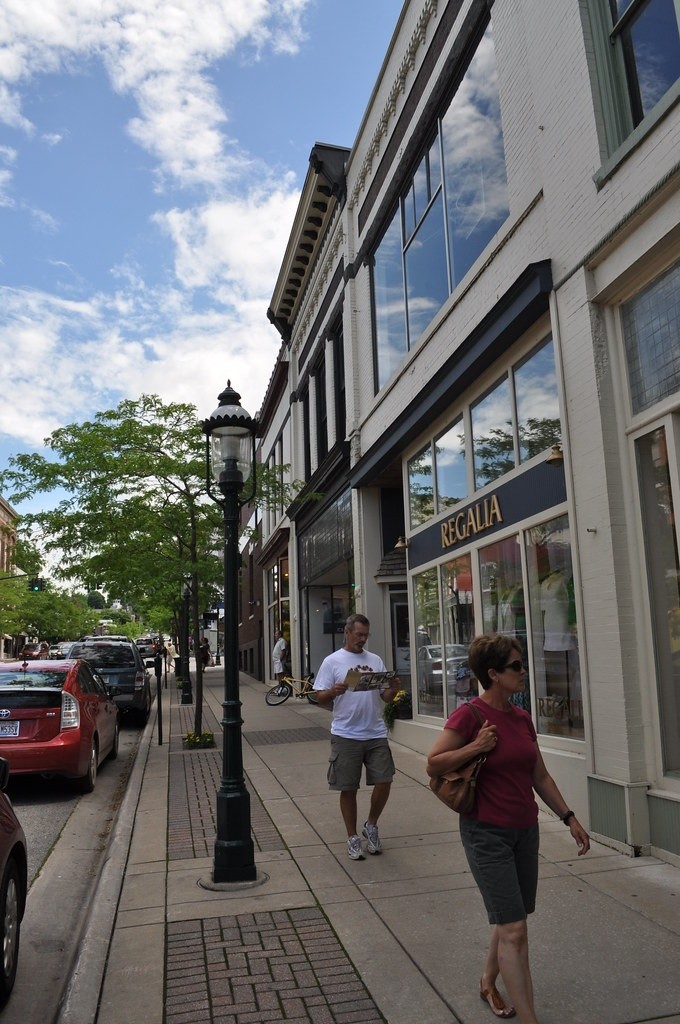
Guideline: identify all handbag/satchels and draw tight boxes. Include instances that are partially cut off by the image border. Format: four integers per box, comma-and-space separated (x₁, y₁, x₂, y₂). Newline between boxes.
429, 703, 488, 814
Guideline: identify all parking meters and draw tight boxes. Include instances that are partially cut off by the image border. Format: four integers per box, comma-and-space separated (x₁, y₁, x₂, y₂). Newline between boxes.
163, 647, 167, 689
154, 653, 162, 745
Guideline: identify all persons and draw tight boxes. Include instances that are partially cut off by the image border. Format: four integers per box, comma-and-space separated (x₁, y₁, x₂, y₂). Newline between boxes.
200, 638, 209, 672
426, 633, 590, 1023
166, 643, 176, 673
496, 585, 525, 637
540, 568, 577, 737
273, 630, 287, 693
313, 614, 402, 860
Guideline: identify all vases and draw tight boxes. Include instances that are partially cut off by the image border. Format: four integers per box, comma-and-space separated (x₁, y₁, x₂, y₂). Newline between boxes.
398, 704, 411, 719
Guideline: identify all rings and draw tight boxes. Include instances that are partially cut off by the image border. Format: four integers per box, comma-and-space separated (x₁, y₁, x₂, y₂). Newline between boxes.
495, 737, 497, 740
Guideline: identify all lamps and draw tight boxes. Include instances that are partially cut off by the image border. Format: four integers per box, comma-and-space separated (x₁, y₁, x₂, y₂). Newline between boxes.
395, 536, 410, 550
545, 443, 564, 467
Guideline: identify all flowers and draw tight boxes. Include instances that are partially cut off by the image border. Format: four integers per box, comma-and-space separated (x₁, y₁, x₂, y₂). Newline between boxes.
384, 690, 412, 734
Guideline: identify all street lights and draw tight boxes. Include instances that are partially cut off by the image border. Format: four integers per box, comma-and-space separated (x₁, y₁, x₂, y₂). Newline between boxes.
199, 376, 261, 884
181, 570, 195, 704
210, 592, 222, 665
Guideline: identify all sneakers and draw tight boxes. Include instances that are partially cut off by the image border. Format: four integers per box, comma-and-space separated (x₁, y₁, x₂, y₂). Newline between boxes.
361, 820, 383, 855
346, 835, 366, 860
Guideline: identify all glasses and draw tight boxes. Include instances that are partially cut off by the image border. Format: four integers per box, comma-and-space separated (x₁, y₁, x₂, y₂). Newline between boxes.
501, 659, 527, 673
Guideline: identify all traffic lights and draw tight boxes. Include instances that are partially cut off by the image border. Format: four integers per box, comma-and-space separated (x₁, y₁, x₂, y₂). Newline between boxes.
84, 577, 102, 594
27, 577, 47, 592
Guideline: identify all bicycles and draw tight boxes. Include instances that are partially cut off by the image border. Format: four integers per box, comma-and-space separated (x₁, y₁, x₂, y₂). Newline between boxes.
265, 672, 318, 706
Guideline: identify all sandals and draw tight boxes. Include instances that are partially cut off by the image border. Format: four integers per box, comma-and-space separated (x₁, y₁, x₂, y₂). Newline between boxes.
479, 976, 515, 1018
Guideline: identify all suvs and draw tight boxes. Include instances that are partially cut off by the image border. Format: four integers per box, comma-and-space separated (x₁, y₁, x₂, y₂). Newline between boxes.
66, 635, 152, 727
19, 641, 49, 659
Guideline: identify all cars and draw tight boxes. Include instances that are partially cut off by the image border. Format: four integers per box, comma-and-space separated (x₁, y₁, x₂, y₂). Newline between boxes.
135, 634, 164, 657
0, 658, 122, 794
0, 758, 27, 1013
49, 642, 77, 658
417, 643, 471, 691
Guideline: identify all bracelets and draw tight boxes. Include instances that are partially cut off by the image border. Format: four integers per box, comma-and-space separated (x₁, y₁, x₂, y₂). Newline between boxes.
563, 811, 574, 826
